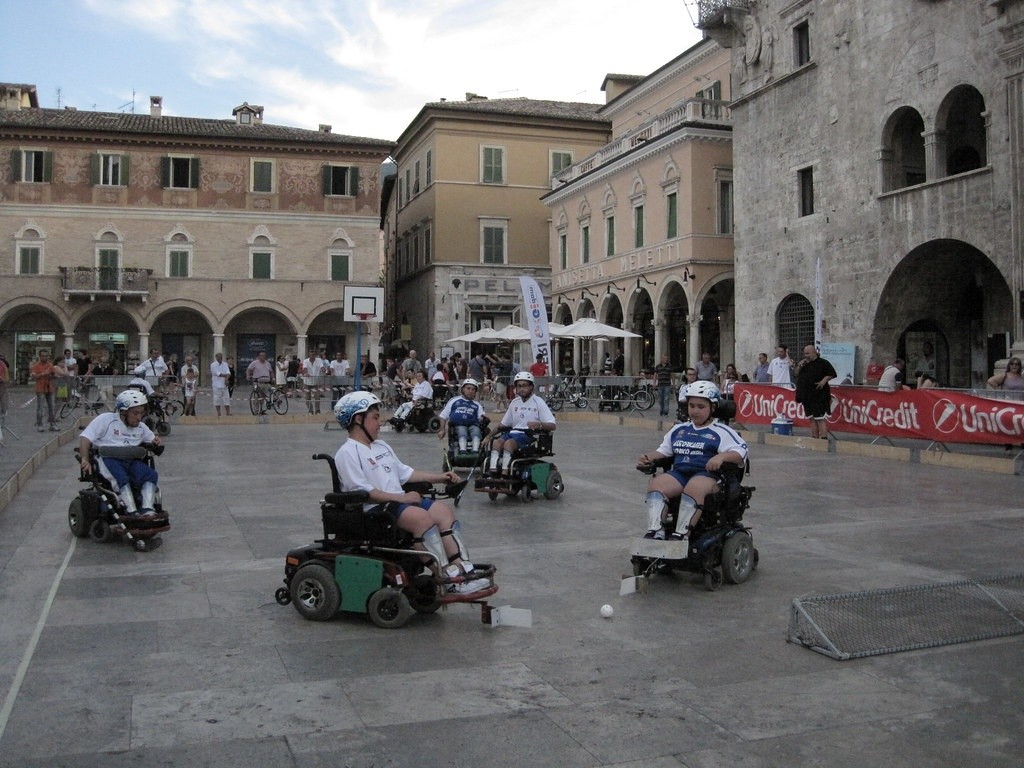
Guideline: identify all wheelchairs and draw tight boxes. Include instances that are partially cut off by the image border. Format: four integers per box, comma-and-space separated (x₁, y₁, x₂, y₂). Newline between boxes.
387, 397, 441, 433
542, 371, 589, 411
128, 384, 171, 437
274, 454, 499, 630
473, 425, 565, 504
618, 432, 760, 597
440, 422, 492, 485
598, 367, 656, 413
431, 378, 458, 410
67, 425, 171, 553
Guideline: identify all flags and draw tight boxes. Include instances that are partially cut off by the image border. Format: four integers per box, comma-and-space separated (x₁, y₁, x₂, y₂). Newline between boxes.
520, 276, 553, 393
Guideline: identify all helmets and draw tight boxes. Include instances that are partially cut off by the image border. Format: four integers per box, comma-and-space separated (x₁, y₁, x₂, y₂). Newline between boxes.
686, 380, 720, 407
133, 366, 146, 374
460, 378, 478, 392
514, 371, 535, 388
333, 391, 382, 429
116, 390, 147, 410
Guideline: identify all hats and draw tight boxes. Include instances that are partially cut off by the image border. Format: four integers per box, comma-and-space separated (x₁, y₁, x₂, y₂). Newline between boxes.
536, 354, 542, 359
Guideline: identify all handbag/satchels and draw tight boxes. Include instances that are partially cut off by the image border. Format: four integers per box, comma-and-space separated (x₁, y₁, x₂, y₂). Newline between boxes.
57, 385, 68, 398
497, 382, 506, 395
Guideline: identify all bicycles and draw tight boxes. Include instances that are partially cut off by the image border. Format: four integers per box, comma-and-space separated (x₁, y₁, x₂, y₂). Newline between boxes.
113, 390, 185, 417
60, 374, 118, 419
247, 375, 289, 416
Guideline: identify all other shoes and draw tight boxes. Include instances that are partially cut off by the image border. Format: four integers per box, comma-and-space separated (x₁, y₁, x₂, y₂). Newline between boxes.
459, 450, 466, 455
473, 451, 479, 456
643, 530, 666, 540
140, 506, 157, 516
396, 421, 404, 431
669, 532, 689, 540
39, 426, 44, 431
500, 474, 509, 481
387, 419, 398, 424
49, 426, 60, 431
487, 472, 496, 479
124, 508, 141, 518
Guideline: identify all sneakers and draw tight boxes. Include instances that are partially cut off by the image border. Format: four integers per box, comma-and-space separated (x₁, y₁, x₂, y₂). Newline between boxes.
442, 564, 484, 594
460, 561, 491, 589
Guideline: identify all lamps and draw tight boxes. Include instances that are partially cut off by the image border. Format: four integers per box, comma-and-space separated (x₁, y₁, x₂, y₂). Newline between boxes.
557, 292, 575, 307
636, 135, 648, 141
559, 178, 568, 184
579, 286, 598, 304
636, 273, 656, 294
683, 266, 696, 286
605, 280, 626, 298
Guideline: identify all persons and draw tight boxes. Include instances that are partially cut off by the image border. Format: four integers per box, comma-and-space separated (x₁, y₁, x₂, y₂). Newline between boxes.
793, 345, 837, 439
987, 358, 1024, 455
29, 347, 235, 432
767, 344, 795, 388
438, 378, 490, 456
752, 353, 772, 382
637, 380, 748, 542
604, 348, 625, 376
876, 359, 910, 392
0, 354, 9, 424
79, 390, 165, 522
916, 371, 940, 390
676, 353, 742, 423
246, 349, 512, 433
652, 355, 675, 417
334, 391, 491, 596
480, 372, 556, 479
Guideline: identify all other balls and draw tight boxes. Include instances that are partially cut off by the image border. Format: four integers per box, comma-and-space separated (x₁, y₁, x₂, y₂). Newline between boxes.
601, 604, 614, 618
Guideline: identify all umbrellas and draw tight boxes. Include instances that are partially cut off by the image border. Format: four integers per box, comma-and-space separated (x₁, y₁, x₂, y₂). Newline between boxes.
443, 317, 642, 371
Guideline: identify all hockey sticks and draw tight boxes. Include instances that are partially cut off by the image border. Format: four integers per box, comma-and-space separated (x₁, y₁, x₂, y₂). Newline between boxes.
87, 471, 164, 552
441, 439, 451, 471
454, 445, 485, 507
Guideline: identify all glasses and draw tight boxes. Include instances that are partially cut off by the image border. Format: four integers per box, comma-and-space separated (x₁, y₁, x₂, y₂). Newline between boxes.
1010, 362, 1020, 366
687, 373, 696, 376
516, 384, 531, 388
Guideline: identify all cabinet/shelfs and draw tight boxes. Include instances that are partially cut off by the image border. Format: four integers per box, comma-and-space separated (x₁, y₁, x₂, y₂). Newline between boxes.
14, 348, 35, 385
